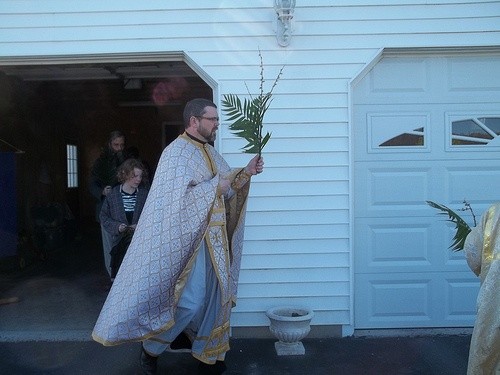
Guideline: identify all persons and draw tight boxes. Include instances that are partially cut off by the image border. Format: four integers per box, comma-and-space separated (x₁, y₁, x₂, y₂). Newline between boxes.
461, 201, 500, 375
90, 128, 134, 228
97, 157, 149, 289
93, 98, 264, 375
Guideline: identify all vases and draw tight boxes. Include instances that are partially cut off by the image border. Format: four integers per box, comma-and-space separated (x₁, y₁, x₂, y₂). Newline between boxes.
265, 305, 313, 355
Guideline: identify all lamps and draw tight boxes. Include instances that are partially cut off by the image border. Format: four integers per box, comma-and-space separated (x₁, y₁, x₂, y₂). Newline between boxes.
270, 0, 298, 46
